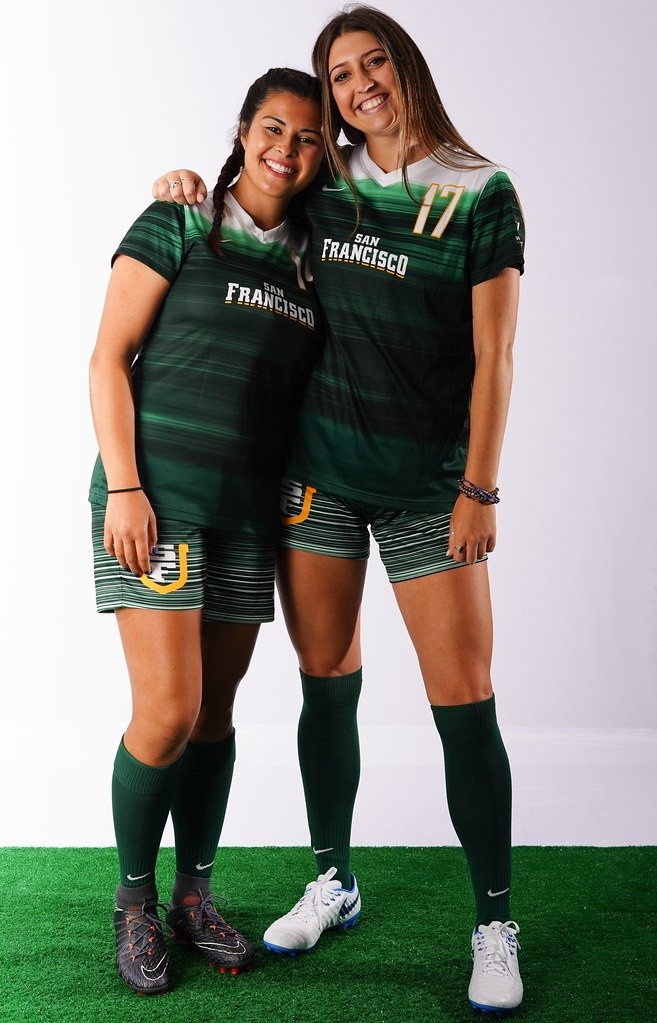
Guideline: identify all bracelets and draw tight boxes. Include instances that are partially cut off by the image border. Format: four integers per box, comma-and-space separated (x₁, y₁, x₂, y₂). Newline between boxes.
459, 478, 501, 506
106, 487, 143, 495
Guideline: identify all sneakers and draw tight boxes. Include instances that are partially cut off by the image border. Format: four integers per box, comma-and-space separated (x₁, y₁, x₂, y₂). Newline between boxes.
167, 871, 252, 974
467, 921, 523, 1010
113, 878, 171, 992
264, 866, 362, 955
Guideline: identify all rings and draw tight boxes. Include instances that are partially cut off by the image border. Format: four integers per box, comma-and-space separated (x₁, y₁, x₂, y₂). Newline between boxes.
449, 532, 454, 535
455, 546, 466, 553
182, 177, 194, 182
169, 181, 182, 188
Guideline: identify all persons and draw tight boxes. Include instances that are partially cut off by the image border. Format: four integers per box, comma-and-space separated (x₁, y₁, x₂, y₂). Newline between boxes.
152, 6, 524, 1007
86, 67, 341, 993
